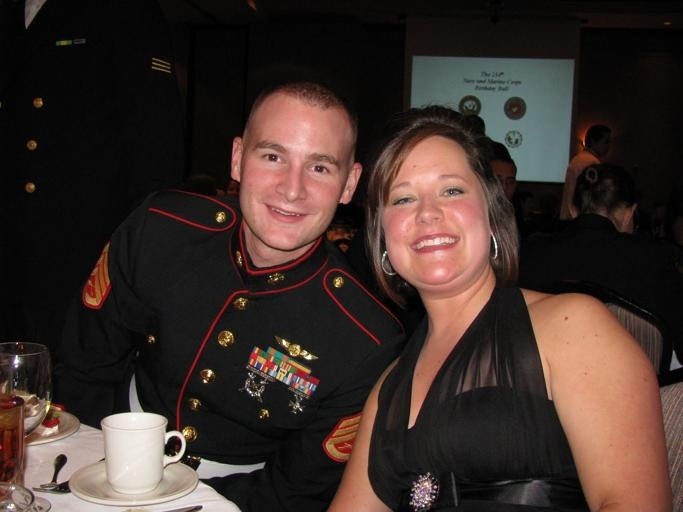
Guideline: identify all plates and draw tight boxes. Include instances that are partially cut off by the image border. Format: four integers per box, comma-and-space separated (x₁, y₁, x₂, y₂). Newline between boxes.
68, 460, 198, 506
23, 410, 81, 447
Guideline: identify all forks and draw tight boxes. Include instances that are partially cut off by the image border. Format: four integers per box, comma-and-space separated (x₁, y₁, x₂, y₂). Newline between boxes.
33, 457, 106, 496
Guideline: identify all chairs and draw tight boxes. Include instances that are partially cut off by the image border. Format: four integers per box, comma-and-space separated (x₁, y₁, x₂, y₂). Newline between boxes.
592, 285, 681, 512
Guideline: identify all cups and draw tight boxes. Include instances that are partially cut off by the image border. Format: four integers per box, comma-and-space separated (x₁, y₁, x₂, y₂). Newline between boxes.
1, 394, 25, 503
100, 411, 187, 494
1, 342, 53, 441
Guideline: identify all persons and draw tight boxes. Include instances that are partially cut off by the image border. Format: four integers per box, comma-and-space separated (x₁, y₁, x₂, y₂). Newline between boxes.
487, 141, 517, 202
315, 100, 674, 512
1, 1, 185, 347
560, 126, 612, 220
519, 161, 673, 323
37, 78, 418, 512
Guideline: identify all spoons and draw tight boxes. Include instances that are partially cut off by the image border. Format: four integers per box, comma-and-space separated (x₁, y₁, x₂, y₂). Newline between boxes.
40, 454, 67, 491
